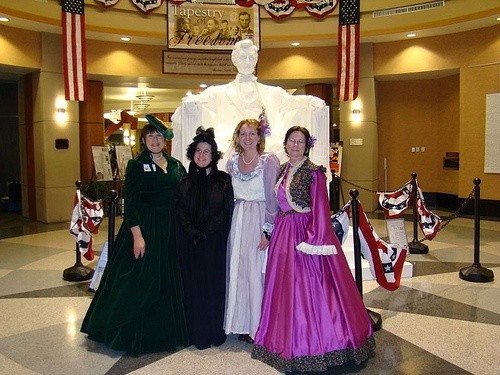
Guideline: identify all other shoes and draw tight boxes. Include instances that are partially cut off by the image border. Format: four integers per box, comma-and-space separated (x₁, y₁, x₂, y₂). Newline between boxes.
238, 334, 254, 343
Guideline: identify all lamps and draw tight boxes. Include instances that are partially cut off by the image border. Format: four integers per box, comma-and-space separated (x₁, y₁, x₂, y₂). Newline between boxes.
55, 96, 67, 125
125, 100, 139, 116
137, 89, 154, 103
135, 101, 150, 111
350, 97, 362, 125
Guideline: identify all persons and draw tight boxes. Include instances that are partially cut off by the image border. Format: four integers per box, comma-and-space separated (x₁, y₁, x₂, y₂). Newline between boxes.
169, 39, 332, 197
202, 12, 254, 40
80, 114, 187, 356
225, 120, 281, 344
251, 125, 375, 373
174, 125, 234, 349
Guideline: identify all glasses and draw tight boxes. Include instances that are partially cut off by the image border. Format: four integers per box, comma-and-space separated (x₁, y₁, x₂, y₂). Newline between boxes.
195, 149, 212, 154
288, 138, 305, 145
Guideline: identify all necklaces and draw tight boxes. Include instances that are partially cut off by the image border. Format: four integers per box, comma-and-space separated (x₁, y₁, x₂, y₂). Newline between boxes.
241, 151, 258, 167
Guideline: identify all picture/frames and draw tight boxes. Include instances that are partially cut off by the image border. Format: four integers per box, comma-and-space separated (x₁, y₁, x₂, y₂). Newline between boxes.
161, 50, 257, 76
166, 1, 261, 49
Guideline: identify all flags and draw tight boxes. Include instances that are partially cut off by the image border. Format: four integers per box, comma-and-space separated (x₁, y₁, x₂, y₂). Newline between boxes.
336, 0, 360, 102
61, 0, 89, 102
